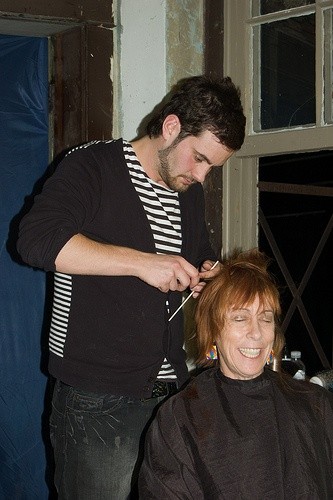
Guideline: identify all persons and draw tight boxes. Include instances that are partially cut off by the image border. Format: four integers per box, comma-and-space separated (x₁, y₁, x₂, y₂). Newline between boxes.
137, 248, 333, 500
16, 72, 247, 500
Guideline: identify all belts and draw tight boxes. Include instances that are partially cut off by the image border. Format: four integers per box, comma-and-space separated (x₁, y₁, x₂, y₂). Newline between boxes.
141, 380, 177, 402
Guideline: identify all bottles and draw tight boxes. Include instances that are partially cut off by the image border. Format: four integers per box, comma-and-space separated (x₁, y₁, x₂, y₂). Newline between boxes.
290, 351, 305, 381
